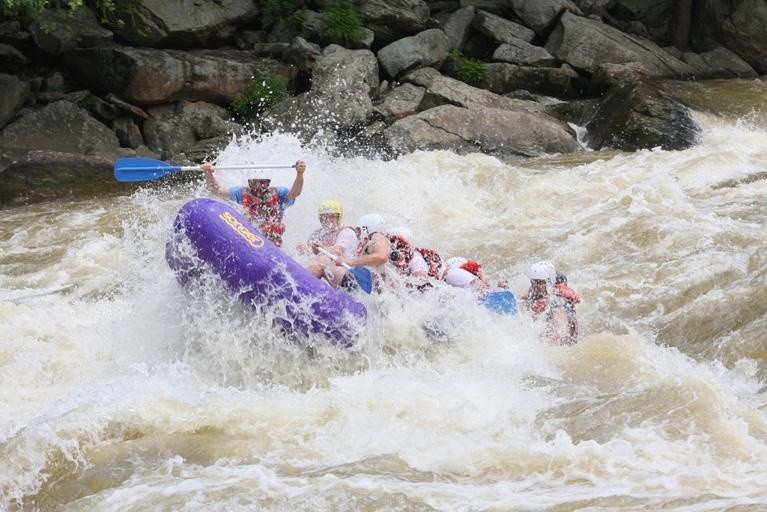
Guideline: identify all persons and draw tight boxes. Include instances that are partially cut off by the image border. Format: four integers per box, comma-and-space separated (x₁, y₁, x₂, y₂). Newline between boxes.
301, 213, 390, 302
201, 158, 307, 248
382, 231, 582, 346
291, 200, 357, 269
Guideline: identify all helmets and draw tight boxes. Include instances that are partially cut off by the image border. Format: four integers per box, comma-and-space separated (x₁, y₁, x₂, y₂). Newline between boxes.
247, 169, 271, 181
317, 199, 344, 225
360, 213, 385, 235
530, 260, 556, 291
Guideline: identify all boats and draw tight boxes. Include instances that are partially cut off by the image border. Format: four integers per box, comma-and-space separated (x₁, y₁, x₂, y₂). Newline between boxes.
164, 198, 578, 349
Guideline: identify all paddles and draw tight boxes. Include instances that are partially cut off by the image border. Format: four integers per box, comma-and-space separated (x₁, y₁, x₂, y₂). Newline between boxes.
478, 291, 517, 316
317, 246, 372, 294
114, 158, 297, 182
